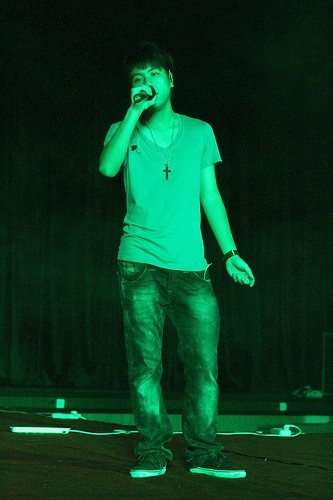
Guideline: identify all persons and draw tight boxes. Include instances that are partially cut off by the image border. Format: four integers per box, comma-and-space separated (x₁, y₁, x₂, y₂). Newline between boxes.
98, 41, 256, 478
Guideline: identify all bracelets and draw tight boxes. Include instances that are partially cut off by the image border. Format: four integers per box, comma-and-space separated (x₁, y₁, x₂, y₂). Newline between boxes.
223, 250, 239, 262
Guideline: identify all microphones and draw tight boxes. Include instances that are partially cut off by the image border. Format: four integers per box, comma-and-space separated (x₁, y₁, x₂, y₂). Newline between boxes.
134, 86, 156, 103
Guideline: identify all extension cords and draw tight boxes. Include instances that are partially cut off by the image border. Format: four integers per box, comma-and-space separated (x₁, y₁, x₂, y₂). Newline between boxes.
270, 427, 292, 436
8, 424, 71, 434
53, 413, 82, 420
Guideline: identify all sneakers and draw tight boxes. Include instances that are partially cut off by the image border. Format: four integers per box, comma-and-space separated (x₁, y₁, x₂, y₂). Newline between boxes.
189, 450, 246, 477
129, 448, 168, 478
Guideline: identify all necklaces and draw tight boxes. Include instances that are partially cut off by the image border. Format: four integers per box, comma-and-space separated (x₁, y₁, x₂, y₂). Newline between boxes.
142, 114, 175, 180
